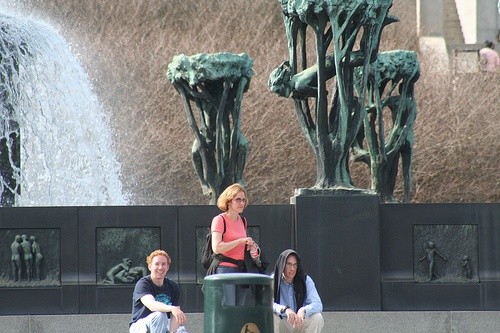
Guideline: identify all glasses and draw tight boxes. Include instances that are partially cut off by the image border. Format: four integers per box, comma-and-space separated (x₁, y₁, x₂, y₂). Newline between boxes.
232, 197, 246, 204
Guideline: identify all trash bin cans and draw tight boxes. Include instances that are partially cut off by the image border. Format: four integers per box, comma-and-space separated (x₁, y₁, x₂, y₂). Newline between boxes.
202, 272, 276, 332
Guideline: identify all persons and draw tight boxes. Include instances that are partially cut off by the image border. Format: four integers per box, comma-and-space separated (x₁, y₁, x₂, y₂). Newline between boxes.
129, 249, 187, 333
102, 257, 150, 285
479, 40, 500, 71
211, 183, 258, 307
10, 234, 43, 283
418, 241, 448, 282
460, 255, 472, 280
270, 248, 324, 333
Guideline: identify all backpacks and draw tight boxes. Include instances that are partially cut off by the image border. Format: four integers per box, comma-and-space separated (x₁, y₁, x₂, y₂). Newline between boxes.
201, 214, 245, 270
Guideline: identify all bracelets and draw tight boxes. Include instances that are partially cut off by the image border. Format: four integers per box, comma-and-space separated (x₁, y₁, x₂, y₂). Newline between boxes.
284, 306, 290, 313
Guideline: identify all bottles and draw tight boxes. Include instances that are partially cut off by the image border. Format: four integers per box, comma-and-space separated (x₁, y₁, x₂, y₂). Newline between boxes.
176, 322, 187, 333
249, 242, 262, 268
